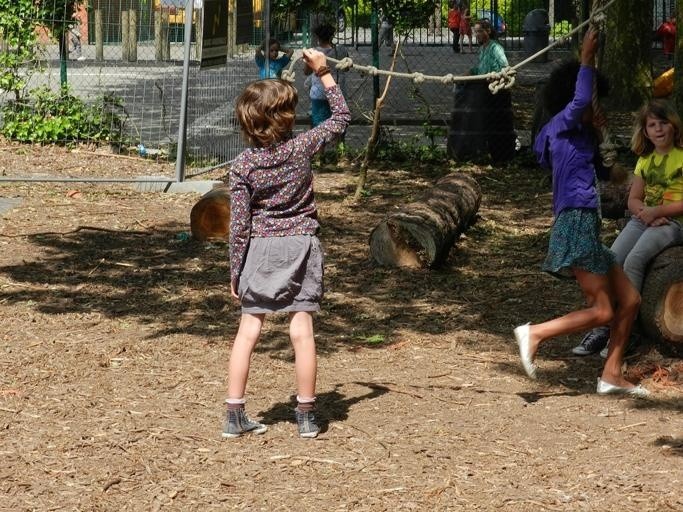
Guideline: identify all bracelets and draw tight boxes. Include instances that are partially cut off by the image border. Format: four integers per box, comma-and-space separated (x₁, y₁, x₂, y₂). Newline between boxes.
315, 65, 331, 77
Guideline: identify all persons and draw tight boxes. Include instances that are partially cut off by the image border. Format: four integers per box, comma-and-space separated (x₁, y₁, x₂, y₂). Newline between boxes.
454, 18, 516, 171
656, 16, 676, 61
255, 39, 294, 80
459, 0, 474, 54
224, 46, 354, 439
68, 13, 85, 62
571, 99, 683, 361
513, 28, 654, 399
448, 0, 460, 53
377, 9, 395, 57
302, 18, 354, 168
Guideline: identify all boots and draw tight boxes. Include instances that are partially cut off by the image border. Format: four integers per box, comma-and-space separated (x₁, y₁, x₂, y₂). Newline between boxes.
222, 402, 267, 438
294, 409, 321, 438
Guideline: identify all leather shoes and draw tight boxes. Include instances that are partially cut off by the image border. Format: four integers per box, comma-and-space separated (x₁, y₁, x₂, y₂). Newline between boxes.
596, 376, 650, 397
512, 320, 538, 380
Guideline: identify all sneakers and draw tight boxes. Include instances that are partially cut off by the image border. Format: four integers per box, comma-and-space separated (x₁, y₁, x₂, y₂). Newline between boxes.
572, 329, 609, 355
599, 333, 638, 359
